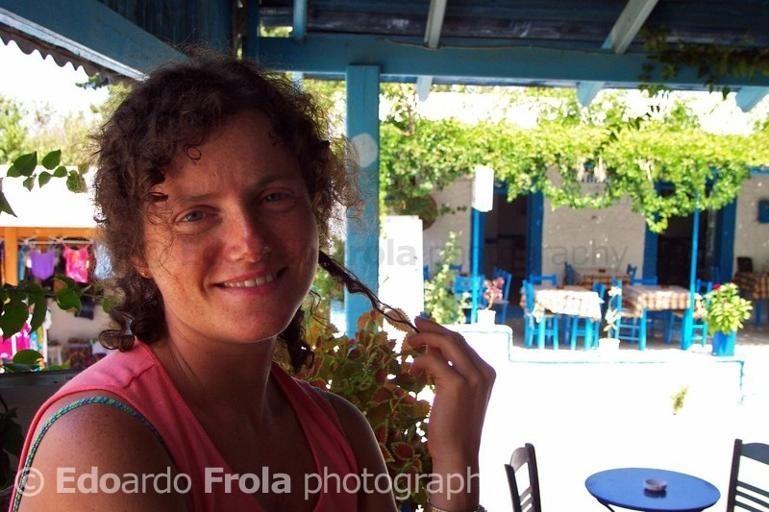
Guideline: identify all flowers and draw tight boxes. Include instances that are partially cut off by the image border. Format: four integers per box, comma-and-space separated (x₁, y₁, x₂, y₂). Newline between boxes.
704, 280, 753, 336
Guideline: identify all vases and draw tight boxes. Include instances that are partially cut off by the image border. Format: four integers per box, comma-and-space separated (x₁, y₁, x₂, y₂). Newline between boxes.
711, 330, 737, 356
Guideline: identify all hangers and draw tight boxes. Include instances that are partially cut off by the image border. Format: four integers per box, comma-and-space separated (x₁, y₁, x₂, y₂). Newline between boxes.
24, 234, 97, 245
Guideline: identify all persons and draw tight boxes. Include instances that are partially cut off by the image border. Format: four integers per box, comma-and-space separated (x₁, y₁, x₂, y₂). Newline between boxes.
9, 47, 496, 512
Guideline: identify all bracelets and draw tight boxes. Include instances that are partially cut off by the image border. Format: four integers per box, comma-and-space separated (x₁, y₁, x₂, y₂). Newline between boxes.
422, 499, 485, 512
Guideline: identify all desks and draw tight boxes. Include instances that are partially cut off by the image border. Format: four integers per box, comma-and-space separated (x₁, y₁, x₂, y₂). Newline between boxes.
586, 469, 720, 512
734, 272, 769, 325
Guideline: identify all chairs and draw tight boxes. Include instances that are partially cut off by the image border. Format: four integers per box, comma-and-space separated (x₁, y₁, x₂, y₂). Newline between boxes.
423, 262, 713, 353
506, 444, 541, 512
726, 438, 769, 512
